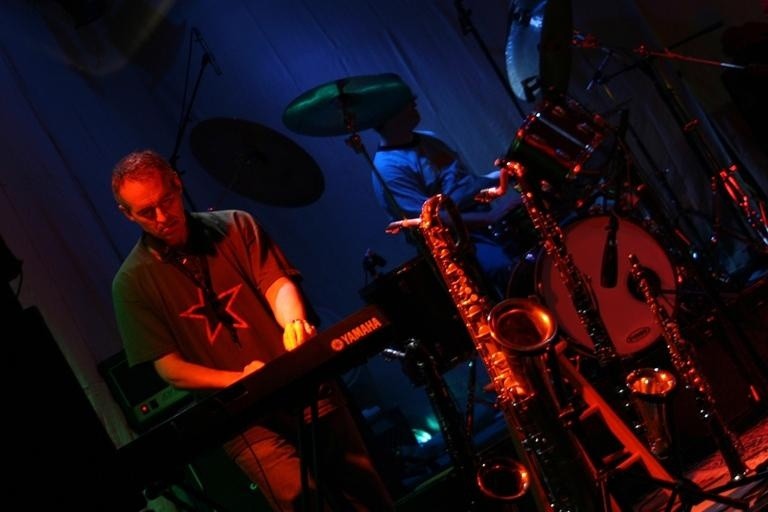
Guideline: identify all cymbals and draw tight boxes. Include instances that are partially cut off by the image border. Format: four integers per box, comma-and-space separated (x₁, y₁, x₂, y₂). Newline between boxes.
282, 74, 412, 136
189, 116, 325, 208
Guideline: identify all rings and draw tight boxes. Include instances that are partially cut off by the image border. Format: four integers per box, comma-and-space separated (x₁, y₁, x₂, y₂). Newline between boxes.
293, 318, 304, 324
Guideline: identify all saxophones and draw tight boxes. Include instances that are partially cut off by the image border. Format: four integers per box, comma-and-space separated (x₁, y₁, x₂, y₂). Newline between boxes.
491, 158, 675, 462
387, 194, 558, 413
383, 338, 532, 512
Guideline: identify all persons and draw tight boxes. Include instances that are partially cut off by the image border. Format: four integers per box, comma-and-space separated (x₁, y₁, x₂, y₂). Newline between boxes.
372, 98, 515, 280
111, 149, 392, 512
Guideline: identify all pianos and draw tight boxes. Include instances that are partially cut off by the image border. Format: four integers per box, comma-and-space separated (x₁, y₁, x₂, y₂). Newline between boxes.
114, 306, 392, 485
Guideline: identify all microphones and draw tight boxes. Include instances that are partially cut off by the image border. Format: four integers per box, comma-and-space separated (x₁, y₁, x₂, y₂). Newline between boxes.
194, 28, 222, 76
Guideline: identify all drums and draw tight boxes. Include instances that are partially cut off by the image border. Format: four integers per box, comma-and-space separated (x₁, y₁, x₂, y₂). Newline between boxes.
509, 83, 605, 194
360, 240, 508, 377
